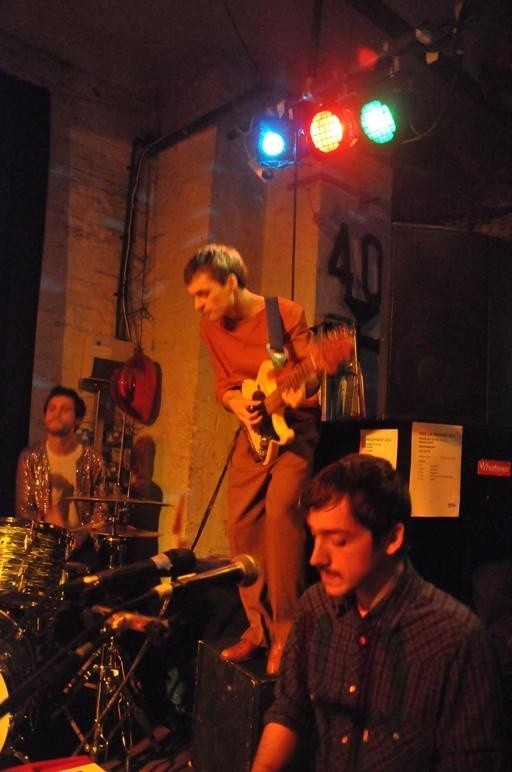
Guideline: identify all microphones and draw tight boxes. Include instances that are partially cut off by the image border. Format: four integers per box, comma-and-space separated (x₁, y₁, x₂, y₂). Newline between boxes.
149, 554, 260, 588
73, 548, 197, 591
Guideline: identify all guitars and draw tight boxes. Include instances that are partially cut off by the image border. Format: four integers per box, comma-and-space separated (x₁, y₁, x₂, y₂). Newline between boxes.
242, 326, 358, 466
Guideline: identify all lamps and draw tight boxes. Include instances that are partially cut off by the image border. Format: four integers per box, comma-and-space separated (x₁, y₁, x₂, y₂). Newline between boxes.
360, 71, 415, 144
254, 114, 300, 170
304, 100, 356, 156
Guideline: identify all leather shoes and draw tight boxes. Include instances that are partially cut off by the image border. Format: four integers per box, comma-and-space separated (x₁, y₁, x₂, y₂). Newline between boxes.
220, 639, 265, 662
266, 639, 285, 677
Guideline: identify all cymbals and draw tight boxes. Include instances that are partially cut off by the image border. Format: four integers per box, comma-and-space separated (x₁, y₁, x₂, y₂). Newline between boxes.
69, 521, 163, 538
66, 496, 170, 505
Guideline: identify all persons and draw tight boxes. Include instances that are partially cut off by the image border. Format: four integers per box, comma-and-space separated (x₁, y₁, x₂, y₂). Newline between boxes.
252, 452, 510, 772
185, 242, 321, 676
15, 384, 110, 577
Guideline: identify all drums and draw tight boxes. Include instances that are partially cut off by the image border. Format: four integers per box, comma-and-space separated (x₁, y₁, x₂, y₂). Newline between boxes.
1, 517, 72, 602
